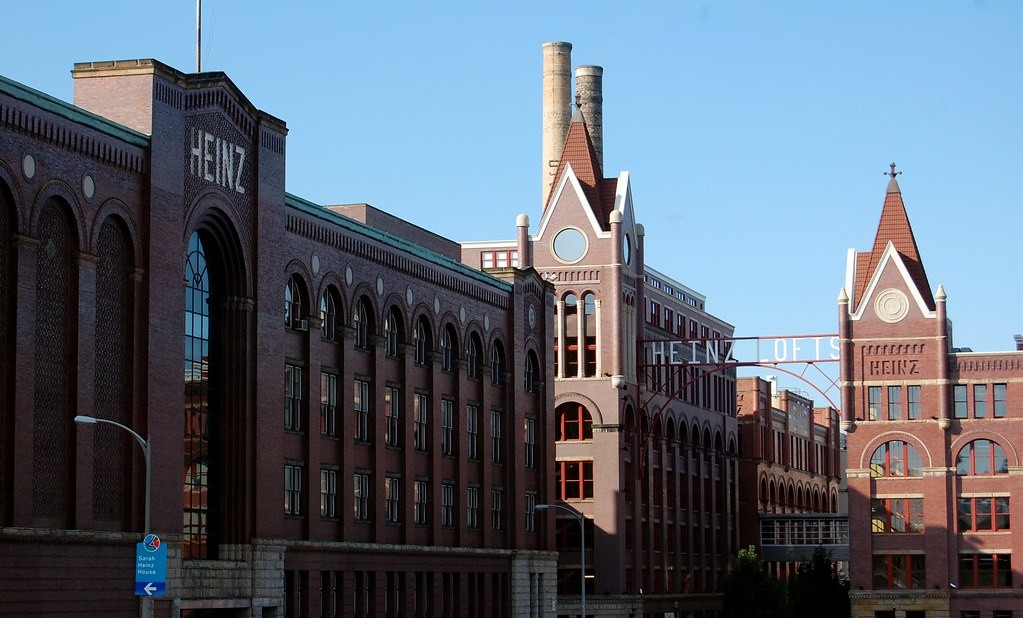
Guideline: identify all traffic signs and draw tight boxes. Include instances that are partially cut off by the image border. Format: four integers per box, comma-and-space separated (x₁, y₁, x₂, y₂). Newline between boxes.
135, 534, 166, 597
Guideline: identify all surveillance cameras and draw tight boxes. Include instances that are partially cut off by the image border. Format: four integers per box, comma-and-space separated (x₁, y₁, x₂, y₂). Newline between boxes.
640, 588, 643, 594
950, 583, 957, 588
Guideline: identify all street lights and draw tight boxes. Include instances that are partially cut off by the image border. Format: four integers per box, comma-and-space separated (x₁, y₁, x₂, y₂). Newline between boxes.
535, 505, 586, 618
75, 415, 156, 618
